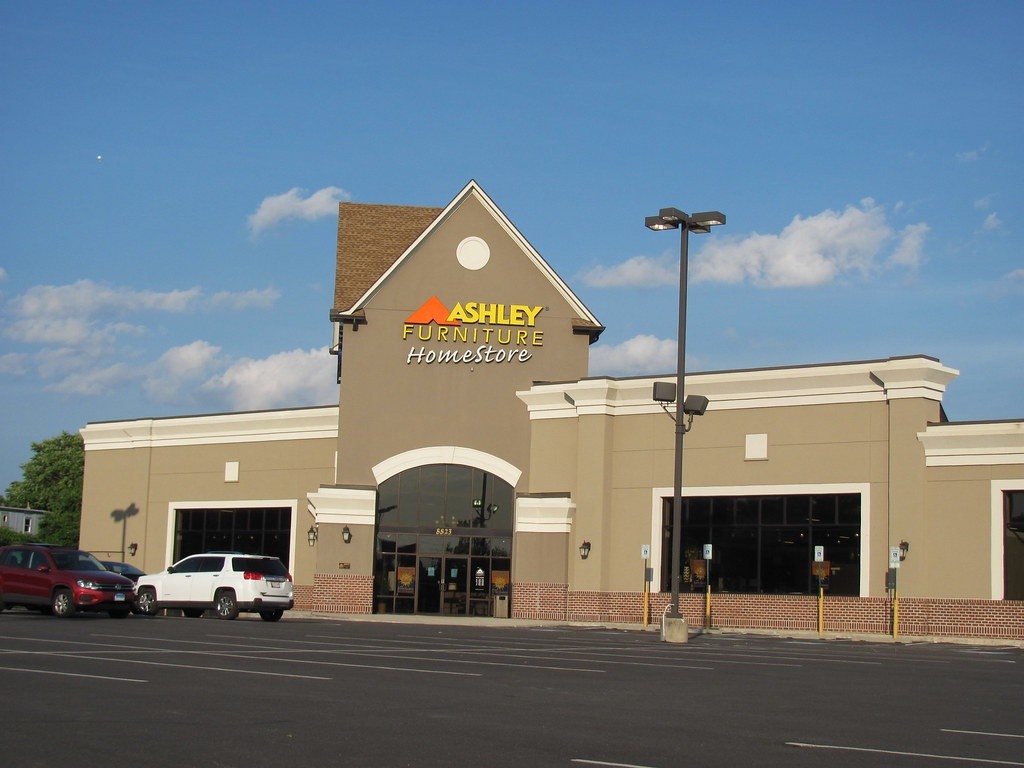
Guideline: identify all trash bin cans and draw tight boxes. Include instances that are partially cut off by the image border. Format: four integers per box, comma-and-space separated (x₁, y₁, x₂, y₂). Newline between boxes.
494, 594, 508, 618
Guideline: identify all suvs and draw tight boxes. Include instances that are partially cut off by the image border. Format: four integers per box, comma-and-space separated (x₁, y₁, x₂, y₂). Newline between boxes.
133, 551, 294, 622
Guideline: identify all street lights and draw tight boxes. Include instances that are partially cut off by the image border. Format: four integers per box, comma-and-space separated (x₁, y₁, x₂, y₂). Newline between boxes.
473, 499, 498, 558
643, 206, 727, 618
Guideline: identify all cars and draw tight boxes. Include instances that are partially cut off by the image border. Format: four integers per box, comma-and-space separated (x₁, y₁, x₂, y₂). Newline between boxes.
76, 560, 147, 615
0, 543, 135, 620
